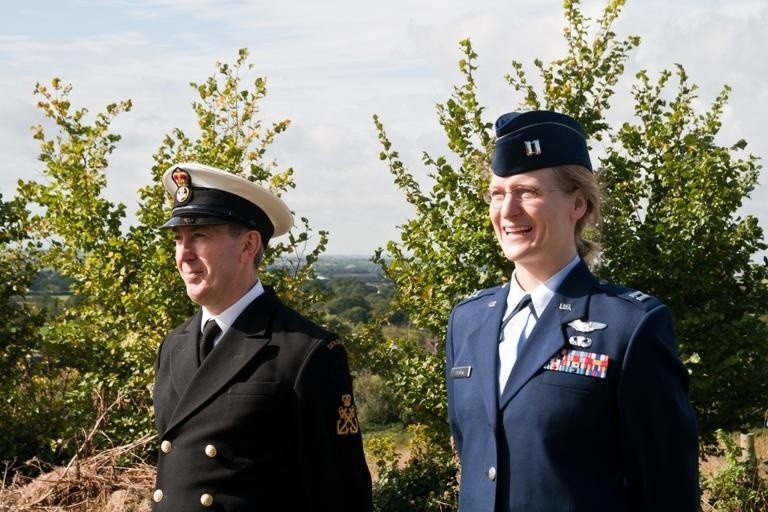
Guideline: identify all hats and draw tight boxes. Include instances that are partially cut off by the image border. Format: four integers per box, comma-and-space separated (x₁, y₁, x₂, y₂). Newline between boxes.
491, 113, 592, 178
158, 163, 294, 246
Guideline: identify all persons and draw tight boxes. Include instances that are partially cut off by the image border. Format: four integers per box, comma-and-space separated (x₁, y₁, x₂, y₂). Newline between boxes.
152, 162, 374, 511
445, 108, 699, 510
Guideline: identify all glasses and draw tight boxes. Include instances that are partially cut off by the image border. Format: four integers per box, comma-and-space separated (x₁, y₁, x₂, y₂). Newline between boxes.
482, 187, 568, 205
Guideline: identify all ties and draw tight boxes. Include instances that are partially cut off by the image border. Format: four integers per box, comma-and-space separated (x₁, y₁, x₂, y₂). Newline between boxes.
198, 317, 221, 360
